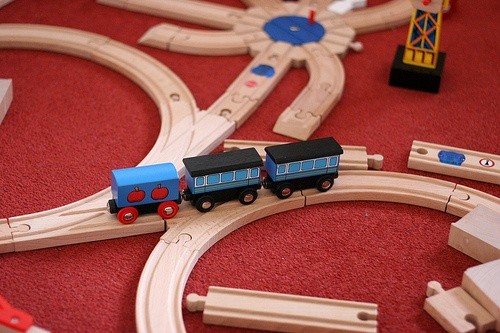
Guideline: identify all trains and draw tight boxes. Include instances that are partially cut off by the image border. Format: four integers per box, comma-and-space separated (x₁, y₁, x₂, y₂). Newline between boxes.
105, 138, 343, 223
